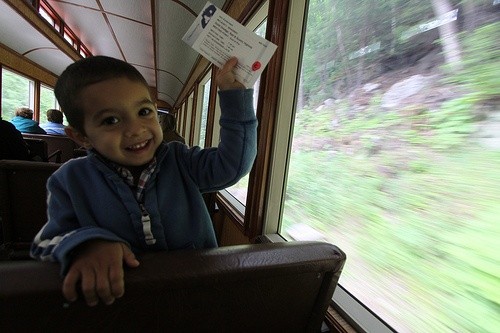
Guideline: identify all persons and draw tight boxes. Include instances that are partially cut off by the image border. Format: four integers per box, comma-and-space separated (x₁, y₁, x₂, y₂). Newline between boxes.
8, 107, 48, 135
29, 55, 258, 305
39, 109, 68, 135
159, 114, 185, 144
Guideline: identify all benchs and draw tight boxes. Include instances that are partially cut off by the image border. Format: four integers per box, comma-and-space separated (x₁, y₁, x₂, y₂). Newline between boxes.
1, 158, 67, 255
157, 110, 186, 144
20, 132, 82, 162
1, 241, 347, 333
